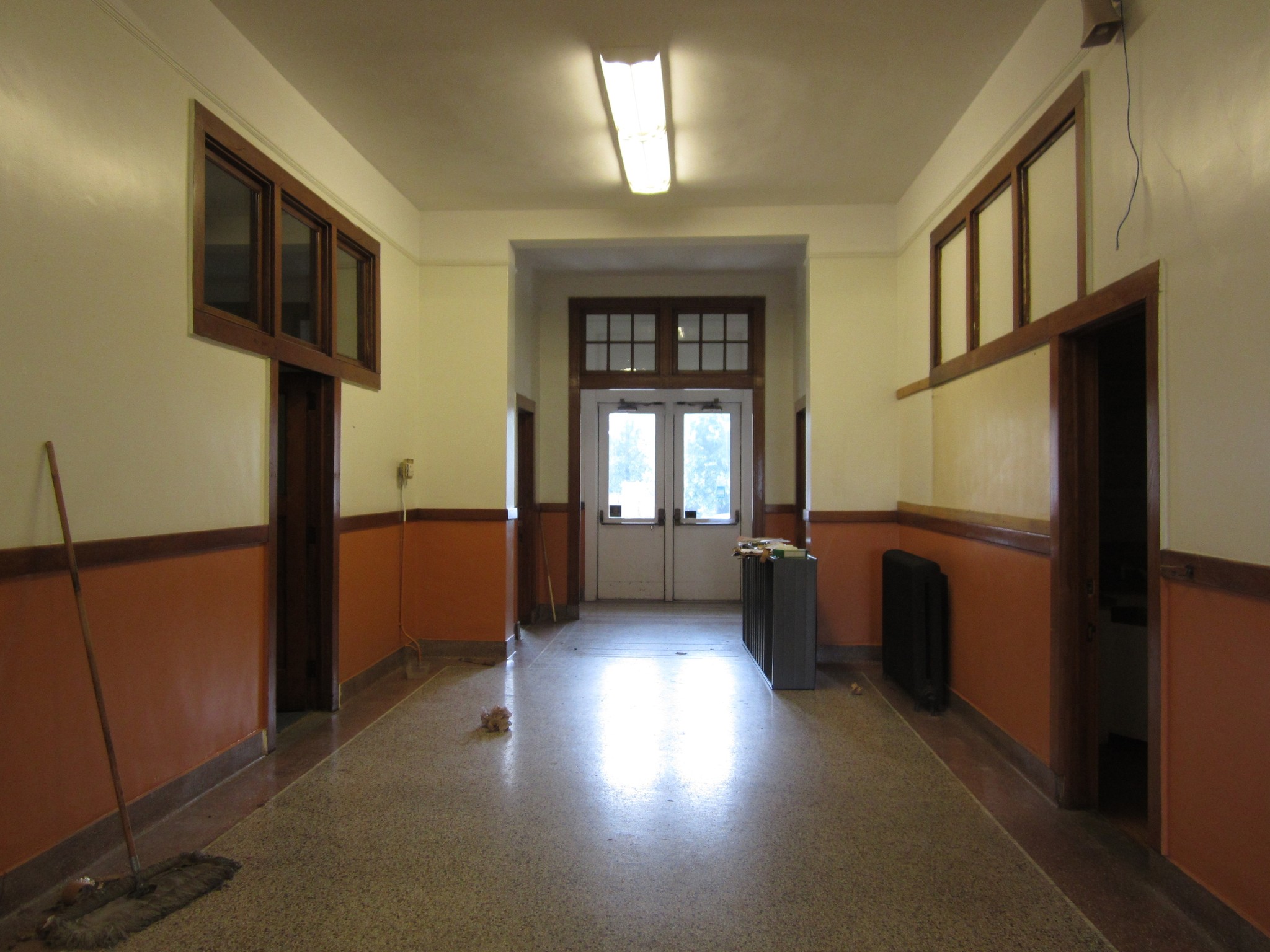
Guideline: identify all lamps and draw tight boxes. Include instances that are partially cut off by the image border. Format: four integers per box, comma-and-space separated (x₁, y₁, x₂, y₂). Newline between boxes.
600, 45, 672, 194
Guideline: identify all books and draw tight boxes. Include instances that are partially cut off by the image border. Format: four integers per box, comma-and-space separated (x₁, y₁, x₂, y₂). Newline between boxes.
773, 549, 807, 559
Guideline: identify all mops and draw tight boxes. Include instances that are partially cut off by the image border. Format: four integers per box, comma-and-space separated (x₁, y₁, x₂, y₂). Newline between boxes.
36, 438, 244, 951
